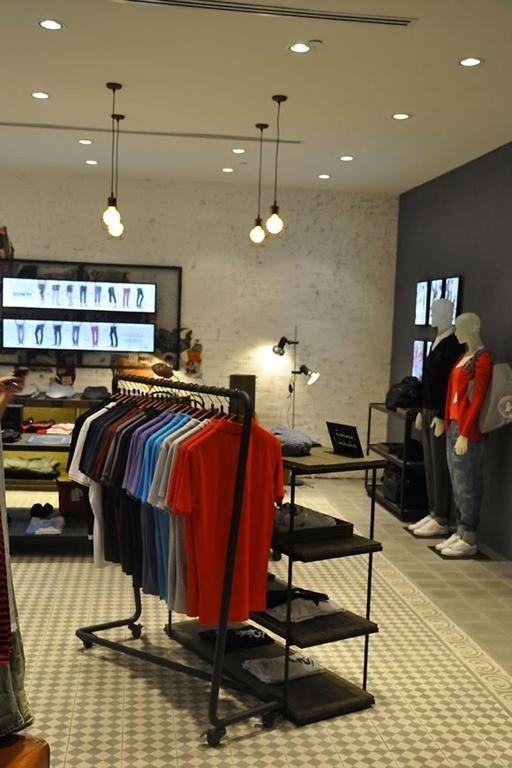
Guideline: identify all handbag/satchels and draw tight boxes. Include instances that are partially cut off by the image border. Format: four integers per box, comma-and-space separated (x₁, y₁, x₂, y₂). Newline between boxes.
466, 348, 512, 435
385, 376, 423, 412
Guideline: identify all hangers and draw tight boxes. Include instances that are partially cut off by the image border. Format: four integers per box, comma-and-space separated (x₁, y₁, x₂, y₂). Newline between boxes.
112, 374, 246, 423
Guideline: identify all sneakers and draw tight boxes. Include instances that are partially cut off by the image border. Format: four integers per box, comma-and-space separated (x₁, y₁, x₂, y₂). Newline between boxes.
407, 514, 450, 537
435, 533, 478, 557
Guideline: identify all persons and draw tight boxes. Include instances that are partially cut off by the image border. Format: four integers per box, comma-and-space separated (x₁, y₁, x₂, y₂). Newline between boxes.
0, 372, 25, 419
408, 298, 467, 536
435, 313, 492, 559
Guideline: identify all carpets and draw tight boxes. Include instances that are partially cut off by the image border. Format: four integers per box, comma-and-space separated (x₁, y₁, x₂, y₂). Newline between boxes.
11, 482, 512, 768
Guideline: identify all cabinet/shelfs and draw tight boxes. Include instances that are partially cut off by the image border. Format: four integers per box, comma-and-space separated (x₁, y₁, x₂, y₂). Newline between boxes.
1, 389, 108, 546
364, 401, 431, 522
166, 446, 386, 726
